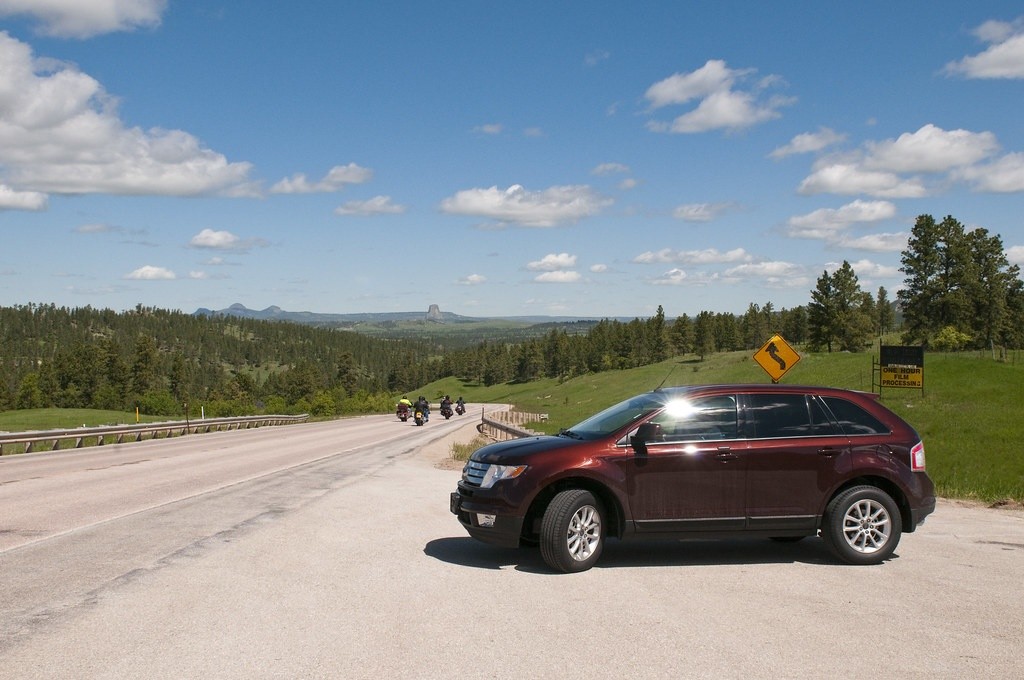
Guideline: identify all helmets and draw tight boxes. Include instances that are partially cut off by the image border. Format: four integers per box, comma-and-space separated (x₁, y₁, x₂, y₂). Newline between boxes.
446, 395, 449, 400
422, 397, 425, 400
460, 397, 462, 400
403, 396, 407, 399
419, 396, 423, 400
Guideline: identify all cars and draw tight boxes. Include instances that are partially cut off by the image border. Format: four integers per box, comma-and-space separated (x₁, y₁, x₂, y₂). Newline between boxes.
450, 385, 939, 575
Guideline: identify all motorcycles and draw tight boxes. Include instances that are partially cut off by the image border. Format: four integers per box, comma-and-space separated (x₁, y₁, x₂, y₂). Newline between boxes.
455, 402, 466, 415
440, 402, 453, 419
415, 409, 430, 425
396, 404, 413, 422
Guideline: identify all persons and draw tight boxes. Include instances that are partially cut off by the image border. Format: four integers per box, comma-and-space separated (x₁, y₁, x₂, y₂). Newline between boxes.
396, 396, 412, 417
413, 396, 430, 421
455, 397, 466, 412
439, 395, 453, 416
660, 401, 725, 439
